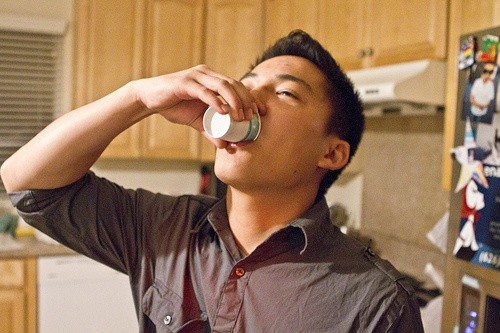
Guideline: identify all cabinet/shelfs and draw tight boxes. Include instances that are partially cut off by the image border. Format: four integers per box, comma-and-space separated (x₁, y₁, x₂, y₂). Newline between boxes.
440, 0, 500, 333
69, 0, 201, 172
199, 0, 266, 164
265, 0, 318, 51
318, 0, 450, 72
0, 256, 38, 333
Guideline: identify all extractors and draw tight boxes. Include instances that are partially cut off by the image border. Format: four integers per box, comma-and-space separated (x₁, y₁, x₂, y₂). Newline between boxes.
346, 58, 446, 116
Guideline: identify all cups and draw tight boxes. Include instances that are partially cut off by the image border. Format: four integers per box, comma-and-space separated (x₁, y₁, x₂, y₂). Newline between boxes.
203, 106, 261, 143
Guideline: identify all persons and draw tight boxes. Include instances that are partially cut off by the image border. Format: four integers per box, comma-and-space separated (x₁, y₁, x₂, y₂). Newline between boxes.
470, 63, 496, 119
1, 30, 425, 333
